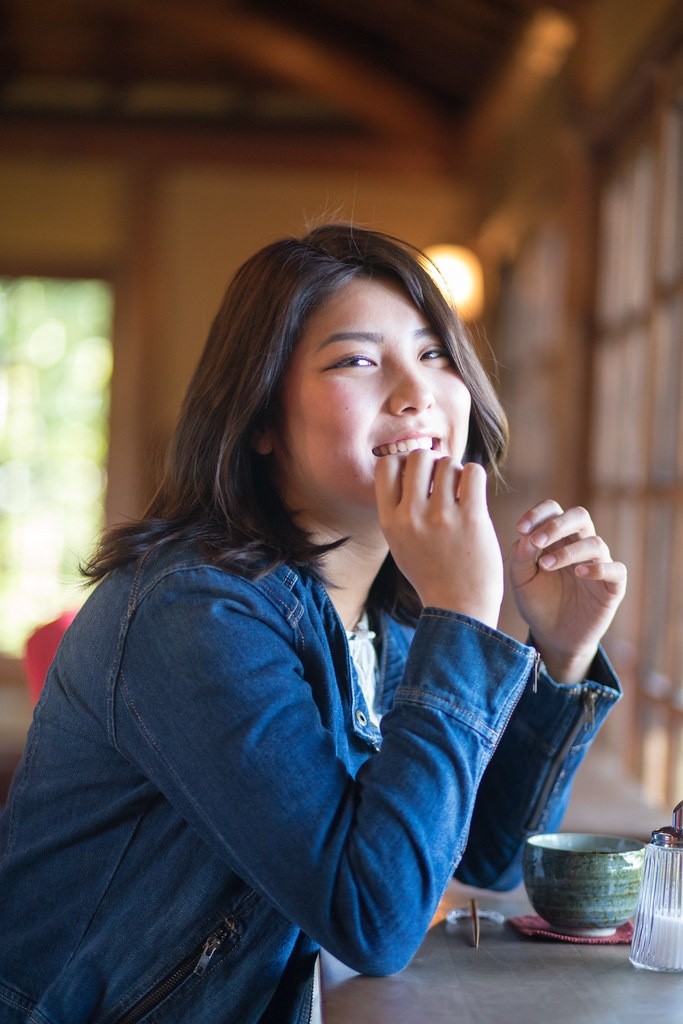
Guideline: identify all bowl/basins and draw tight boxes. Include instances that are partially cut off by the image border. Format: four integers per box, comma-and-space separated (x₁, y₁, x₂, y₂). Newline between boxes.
523, 833, 647, 936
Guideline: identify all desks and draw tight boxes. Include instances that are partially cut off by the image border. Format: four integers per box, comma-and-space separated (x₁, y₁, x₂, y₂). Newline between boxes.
320, 904, 683, 1024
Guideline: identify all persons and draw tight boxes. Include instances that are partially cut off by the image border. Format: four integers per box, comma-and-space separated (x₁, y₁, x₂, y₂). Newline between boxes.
0, 225, 627, 1024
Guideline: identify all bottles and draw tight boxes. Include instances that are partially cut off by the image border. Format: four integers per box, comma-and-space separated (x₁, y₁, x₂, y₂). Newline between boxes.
629, 800, 683, 972
